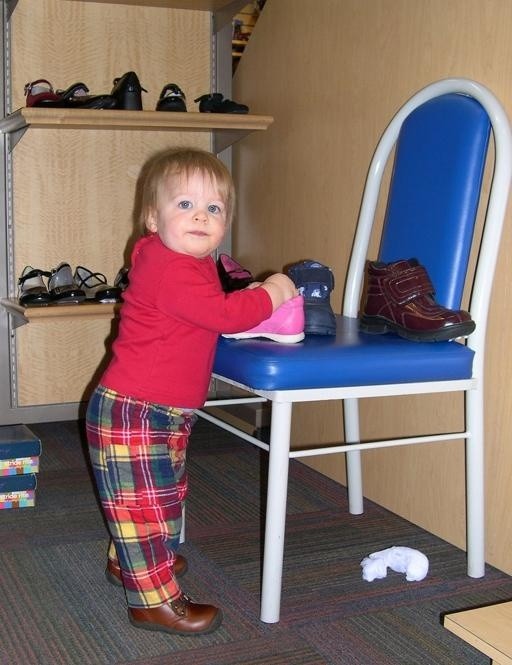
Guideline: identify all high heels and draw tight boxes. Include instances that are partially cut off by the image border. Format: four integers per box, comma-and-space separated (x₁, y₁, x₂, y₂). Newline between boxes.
112, 71, 143, 110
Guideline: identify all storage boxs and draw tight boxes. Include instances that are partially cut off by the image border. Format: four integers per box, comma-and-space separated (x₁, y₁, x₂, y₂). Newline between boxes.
0, 425, 42, 510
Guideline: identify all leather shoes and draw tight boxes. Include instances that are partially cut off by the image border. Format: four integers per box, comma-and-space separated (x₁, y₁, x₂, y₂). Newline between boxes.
156, 83, 187, 110
106, 554, 186, 588
18, 261, 128, 306
217, 253, 254, 289
194, 92, 248, 113
128, 594, 221, 636
24, 79, 113, 109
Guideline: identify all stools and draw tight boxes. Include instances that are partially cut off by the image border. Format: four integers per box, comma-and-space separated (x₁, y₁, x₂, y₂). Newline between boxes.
443, 601, 512, 665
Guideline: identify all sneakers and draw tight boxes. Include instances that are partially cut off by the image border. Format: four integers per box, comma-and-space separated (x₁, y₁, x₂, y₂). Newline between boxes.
222, 295, 307, 343
359, 258, 474, 342
288, 260, 335, 337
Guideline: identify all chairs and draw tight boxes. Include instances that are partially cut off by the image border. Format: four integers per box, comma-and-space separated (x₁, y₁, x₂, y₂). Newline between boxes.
212, 76, 512, 624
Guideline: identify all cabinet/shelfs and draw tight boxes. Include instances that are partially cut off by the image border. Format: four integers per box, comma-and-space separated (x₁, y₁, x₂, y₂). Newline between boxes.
4, 107, 273, 332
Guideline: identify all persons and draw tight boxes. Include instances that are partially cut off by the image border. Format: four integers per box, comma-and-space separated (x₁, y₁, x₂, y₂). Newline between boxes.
78, 147, 298, 639
